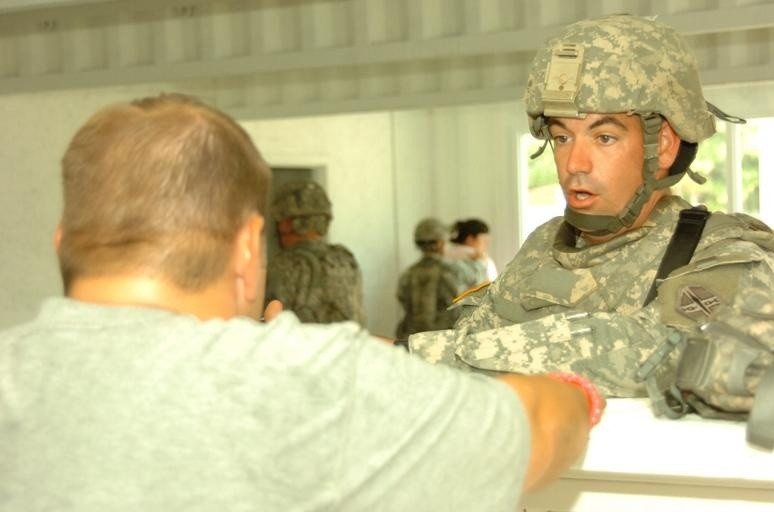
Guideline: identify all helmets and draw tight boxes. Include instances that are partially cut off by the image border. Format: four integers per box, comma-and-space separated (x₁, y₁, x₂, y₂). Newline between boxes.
269, 179, 333, 223
524, 12, 718, 143
414, 218, 449, 241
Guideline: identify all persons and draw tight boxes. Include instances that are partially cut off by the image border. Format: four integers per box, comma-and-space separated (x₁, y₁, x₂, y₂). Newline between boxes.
0, 93, 589, 512
407, 14, 774, 421
396, 217, 459, 347
267, 176, 365, 324
449, 219, 498, 296
